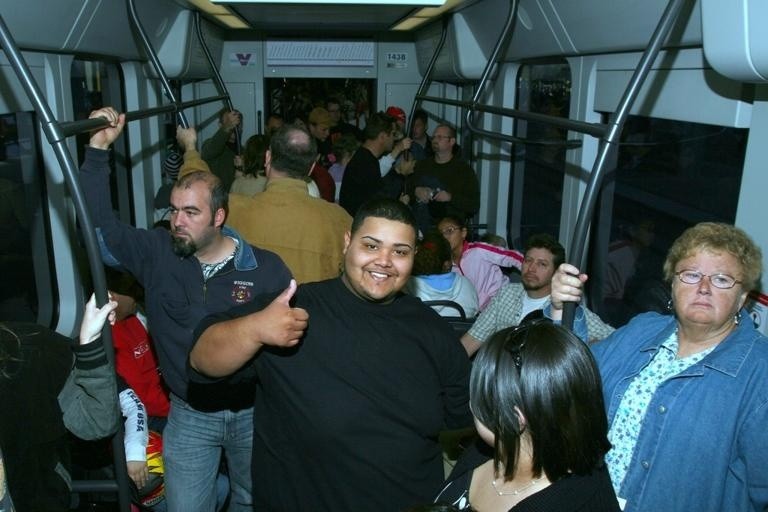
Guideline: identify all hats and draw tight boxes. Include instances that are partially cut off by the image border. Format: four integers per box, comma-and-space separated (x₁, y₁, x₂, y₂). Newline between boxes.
385, 107, 405, 124
309, 107, 337, 127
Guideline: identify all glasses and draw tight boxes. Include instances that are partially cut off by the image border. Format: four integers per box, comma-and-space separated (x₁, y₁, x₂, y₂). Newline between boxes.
432, 135, 449, 140
442, 226, 461, 234
504, 318, 554, 377
674, 269, 742, 289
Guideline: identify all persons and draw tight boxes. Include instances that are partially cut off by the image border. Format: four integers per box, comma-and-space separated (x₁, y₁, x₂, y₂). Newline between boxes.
0, 99, 768, 512
